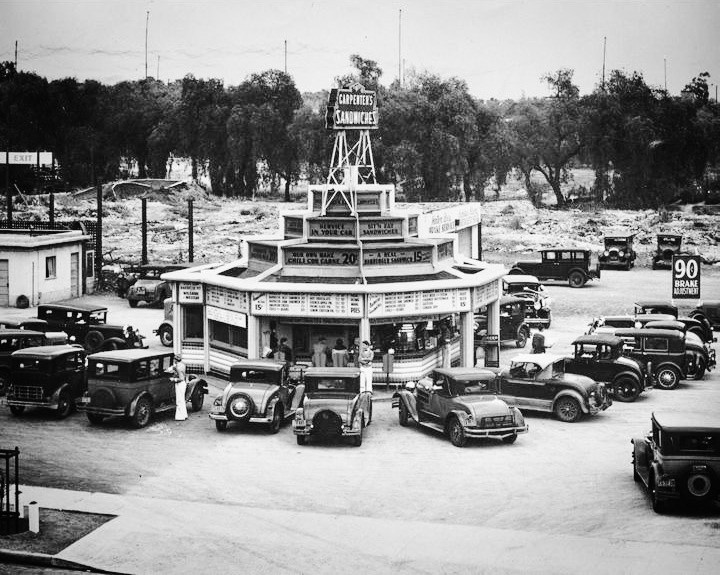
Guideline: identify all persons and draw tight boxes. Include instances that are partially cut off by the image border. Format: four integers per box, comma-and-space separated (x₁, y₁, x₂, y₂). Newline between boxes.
527, 285, 553, 321
358, 341, 374, 394
439, 321, 450, 346
269, 332, 349, 367
532, 324, 552, 353
169, 355, 188, 420
414, 322, 428, 340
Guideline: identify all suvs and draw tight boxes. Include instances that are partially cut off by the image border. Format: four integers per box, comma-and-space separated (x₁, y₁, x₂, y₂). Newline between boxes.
596, 233, 638, 271
36, 302, 150, 351
583, 300, 720, 389
208, 360, 308, 433
651, 231, 684, 271
542, 335, 659, 403
152, 297, 174, 347
506, 248, 602, 288
487, 354, 615, 423
473, 296, 532, 349
292, 366, 374, 446
0, 344, 104, 419
83, 349, 210, 428
627, 410, 720, 514
500, 275, 554, 331
0, 318, 72, 398
391, 368, 529, 447
126, 265, 177, 310
